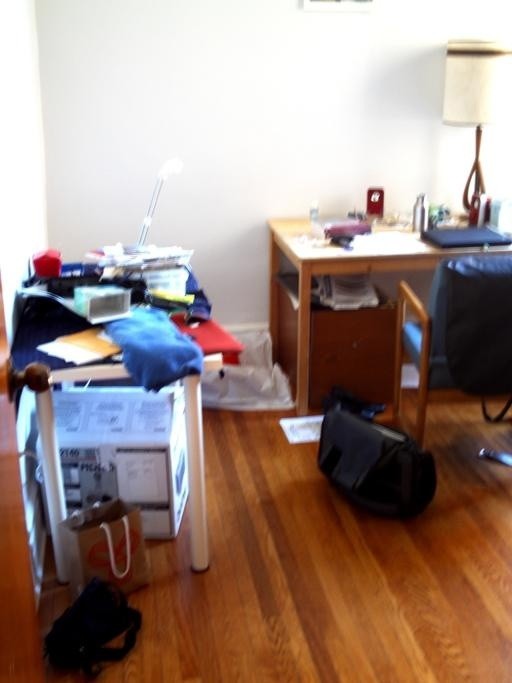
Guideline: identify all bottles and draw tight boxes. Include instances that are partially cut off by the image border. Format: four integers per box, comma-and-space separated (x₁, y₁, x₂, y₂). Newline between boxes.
412, 192, 428, 233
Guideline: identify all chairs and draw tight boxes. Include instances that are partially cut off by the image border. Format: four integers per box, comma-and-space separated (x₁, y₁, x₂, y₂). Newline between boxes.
392, 252, 512, 448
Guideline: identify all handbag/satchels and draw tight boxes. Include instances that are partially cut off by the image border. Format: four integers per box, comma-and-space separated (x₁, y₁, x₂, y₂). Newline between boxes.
57, 499, 151, 600
316, 399, 436, 521
42, 577, 142, 681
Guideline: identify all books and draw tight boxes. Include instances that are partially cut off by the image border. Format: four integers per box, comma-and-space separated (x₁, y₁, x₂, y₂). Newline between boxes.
279, 414, 325, 444
317, 273, 379, 311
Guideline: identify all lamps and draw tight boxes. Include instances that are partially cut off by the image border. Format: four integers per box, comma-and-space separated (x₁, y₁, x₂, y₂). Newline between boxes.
441, 37, 512, 226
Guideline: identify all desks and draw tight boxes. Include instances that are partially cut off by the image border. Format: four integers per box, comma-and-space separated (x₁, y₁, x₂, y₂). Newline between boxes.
10, 243, 223, 582
267, 213, 512, 418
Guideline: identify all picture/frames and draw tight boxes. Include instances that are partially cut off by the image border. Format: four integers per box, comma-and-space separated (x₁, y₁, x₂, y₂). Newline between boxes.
298, 0, 373, 12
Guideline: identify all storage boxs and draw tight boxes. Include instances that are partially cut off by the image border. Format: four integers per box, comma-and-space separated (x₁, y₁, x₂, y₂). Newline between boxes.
278, 273, 396, 409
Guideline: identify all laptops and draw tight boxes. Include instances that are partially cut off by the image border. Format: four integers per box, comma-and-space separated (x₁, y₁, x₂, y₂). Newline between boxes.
420, 225, 512, 248
